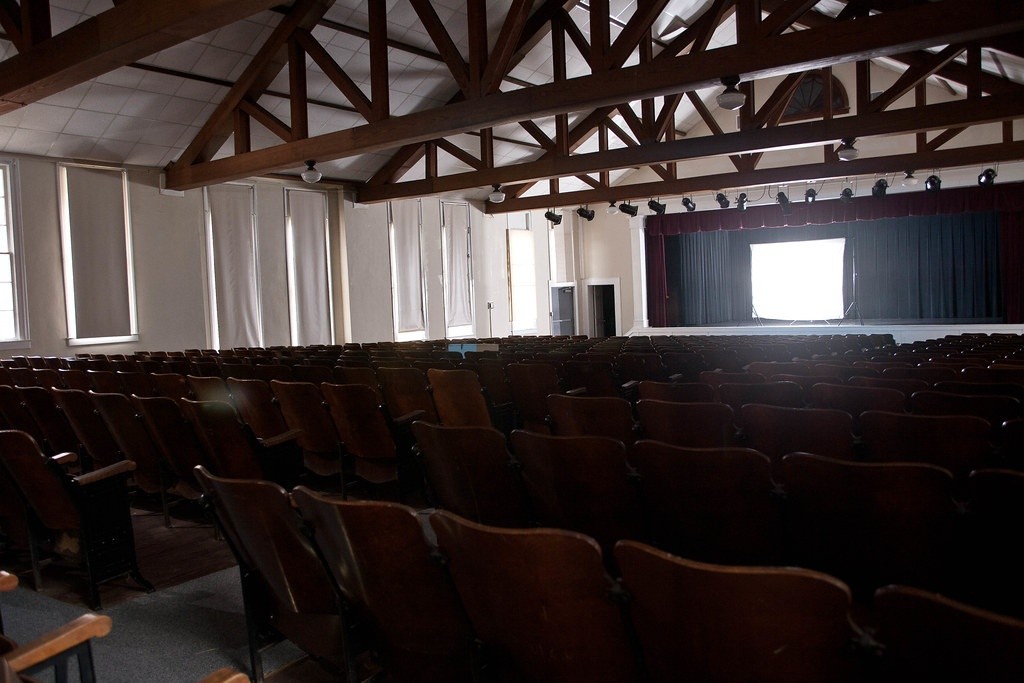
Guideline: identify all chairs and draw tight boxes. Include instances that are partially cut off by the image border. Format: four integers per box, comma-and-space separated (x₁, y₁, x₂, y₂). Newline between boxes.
0, 334, 1024, 683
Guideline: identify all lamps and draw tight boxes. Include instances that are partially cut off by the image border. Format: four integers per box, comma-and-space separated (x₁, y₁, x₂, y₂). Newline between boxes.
648, 196, 665, 215
978, 168, 997, 185
714, 191, 729, 208
804, 189, 817, 204
837, 137, 857, 162
301, 159, 322, 184
607, 201, 620, 215
873, 178, 888, 197
489, 183, 506, 203
736, 189, 749, 213
545, 207, 562, 225
619, 199, 638, 217
577, 203, 595, 221
926, 176, 941, 192
775, 184, 793, 212
682, 194, 696, 211
901, 170, 920, 188
716, 75, 746, 109
